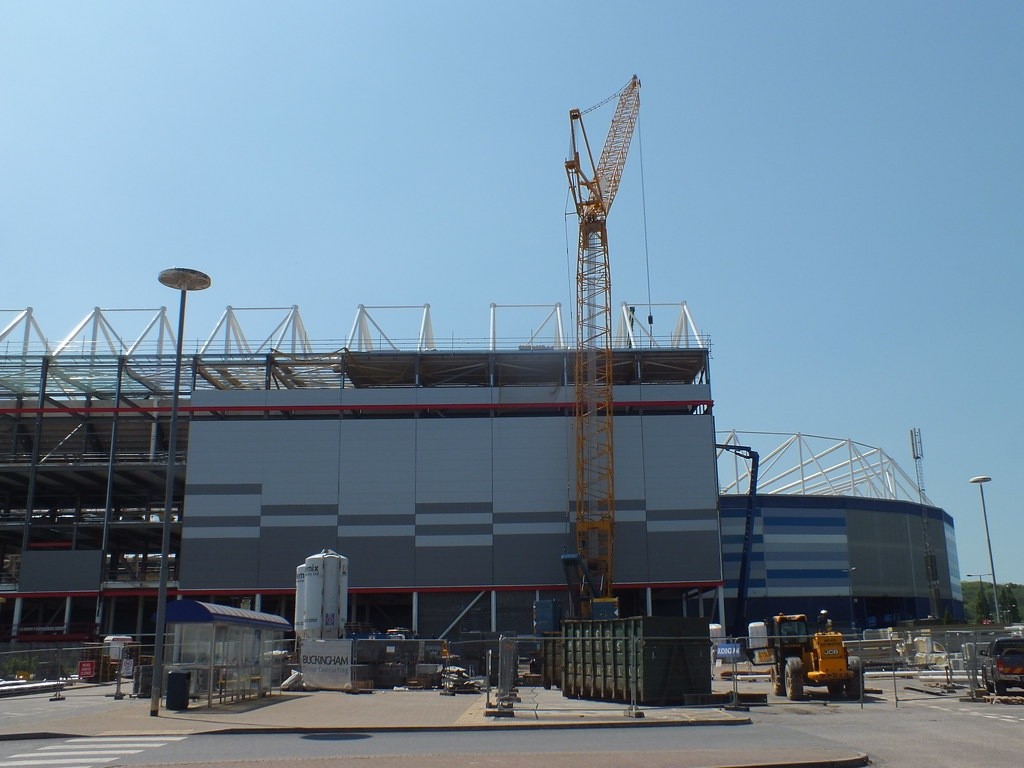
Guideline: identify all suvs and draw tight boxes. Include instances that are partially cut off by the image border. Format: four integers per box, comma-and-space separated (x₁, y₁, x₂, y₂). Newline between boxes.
978, 633, 1024, 696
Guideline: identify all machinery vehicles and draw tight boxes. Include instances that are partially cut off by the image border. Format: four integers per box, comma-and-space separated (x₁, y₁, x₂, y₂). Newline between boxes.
756, 609, 864, 702
559, 75, 644, 628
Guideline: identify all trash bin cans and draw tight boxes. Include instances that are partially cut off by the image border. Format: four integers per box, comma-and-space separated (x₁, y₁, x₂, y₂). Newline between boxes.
166, 670, 191, 711
133, 664, 164, 698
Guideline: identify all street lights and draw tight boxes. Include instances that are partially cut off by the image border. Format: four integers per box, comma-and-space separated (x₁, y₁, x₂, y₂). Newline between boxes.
970, 476, 1001, 623
149, 267, 216, 716
842, 567, 858, 632
967, 573, 992, 618
